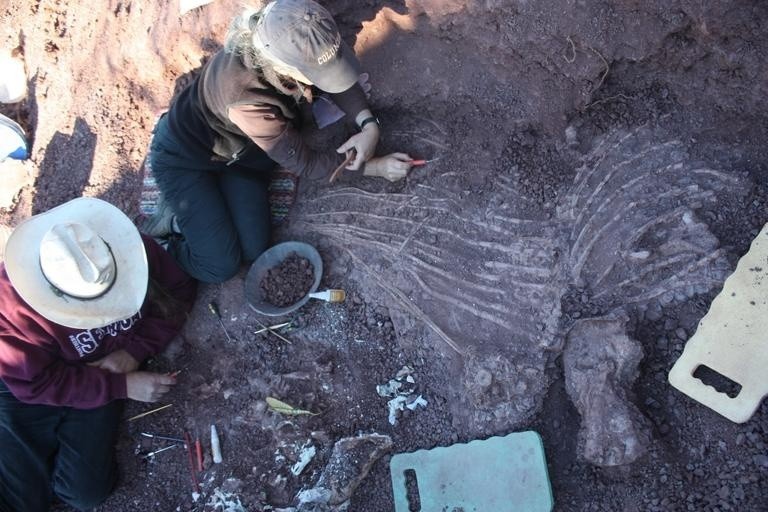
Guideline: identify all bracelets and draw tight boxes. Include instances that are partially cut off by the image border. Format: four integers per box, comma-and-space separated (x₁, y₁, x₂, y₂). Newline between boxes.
359, 116, 380, 130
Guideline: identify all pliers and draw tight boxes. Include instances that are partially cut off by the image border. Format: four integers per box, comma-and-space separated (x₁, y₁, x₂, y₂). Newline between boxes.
280, 312, 309, 334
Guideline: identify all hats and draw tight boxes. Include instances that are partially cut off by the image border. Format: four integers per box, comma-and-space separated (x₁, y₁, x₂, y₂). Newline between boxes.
3, 197, 149, 330
259, 1, 360, 95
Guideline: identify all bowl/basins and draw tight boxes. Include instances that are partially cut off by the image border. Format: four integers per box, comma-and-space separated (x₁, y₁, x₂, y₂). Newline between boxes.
242, 238, 326, 320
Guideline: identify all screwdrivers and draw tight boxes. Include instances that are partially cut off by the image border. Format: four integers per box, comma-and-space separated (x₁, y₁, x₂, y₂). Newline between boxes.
208, 302, 231, 342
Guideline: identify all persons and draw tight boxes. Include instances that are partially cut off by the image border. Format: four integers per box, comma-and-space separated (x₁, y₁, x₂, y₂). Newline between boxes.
0, 192, 197, 511
129, 1, 415, 286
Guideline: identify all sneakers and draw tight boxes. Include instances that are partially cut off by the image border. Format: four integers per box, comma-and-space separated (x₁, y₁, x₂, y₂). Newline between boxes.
140, 195, 176, 240
